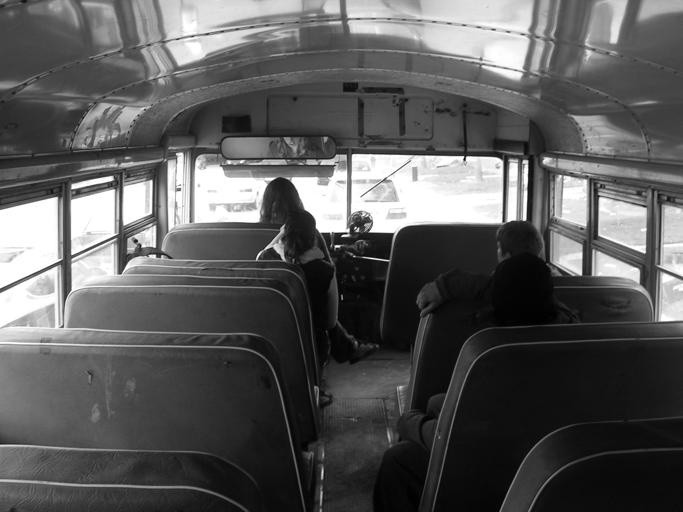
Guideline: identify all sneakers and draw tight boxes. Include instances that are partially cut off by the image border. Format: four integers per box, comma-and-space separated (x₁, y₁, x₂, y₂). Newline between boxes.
349, 343, 380, 364
319, 392, 333, 406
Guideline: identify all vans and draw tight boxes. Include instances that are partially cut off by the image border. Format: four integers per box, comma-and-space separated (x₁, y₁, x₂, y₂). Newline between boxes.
317, 171, 410, 233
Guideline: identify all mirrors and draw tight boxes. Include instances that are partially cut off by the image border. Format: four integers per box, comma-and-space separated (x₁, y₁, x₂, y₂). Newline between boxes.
220, 134, 337, 160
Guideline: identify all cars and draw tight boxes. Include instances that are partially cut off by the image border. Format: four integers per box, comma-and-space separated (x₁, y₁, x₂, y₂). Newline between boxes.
206, 170, 267, 212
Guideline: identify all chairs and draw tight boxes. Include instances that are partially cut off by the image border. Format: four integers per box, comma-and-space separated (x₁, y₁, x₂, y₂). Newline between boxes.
496, 415, 682, 512
0, 442, 264, 512
121, 256, 324, 393
161, 220, 285, 263
62, 275, 320, 441
378, 221, 505, 347
414, 319, 683, 511
0, 325, 314, 512
392, 275, 654, 421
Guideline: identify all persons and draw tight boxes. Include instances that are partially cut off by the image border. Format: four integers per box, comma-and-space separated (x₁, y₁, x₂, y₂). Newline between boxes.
266, 137, 324, 159
489, 221, 561, 276
372, 254, 580, 512
260, 177, 380, 365
256, 211, 338, 408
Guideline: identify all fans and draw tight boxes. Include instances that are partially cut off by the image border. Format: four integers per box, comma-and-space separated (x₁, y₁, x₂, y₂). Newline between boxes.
347, 210, 373, 255
